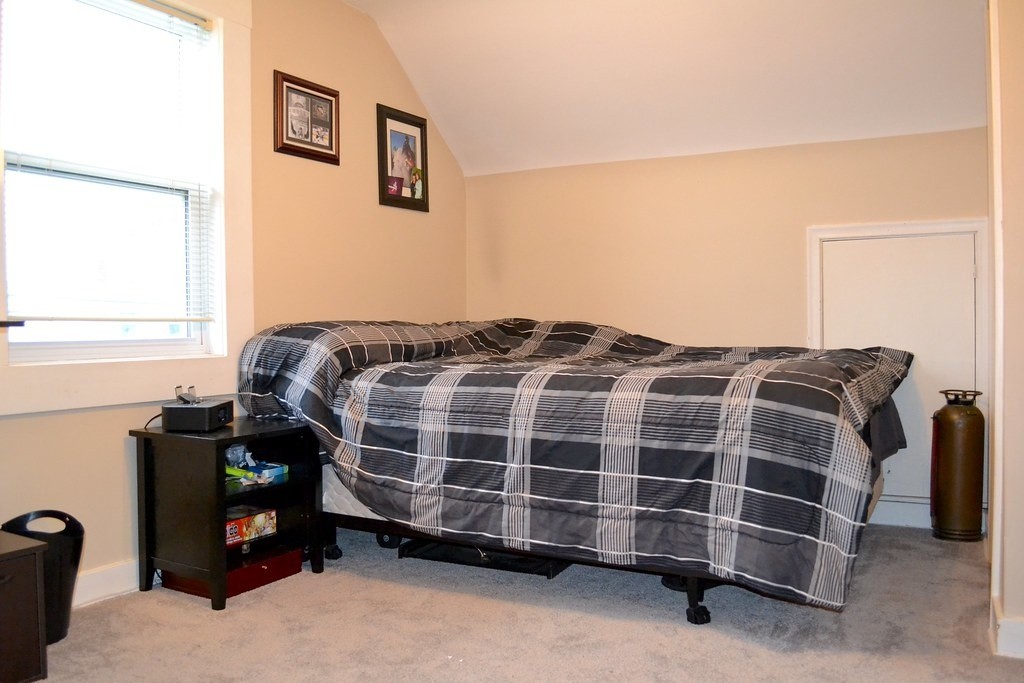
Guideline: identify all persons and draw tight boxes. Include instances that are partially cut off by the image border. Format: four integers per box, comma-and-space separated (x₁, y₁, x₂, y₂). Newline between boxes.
393, 136, 416, 187
389, 181, 397, 194
411, 171, 423, 199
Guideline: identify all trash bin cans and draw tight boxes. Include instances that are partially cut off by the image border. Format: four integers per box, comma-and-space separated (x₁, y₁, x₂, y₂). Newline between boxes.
1, 510, 85, 646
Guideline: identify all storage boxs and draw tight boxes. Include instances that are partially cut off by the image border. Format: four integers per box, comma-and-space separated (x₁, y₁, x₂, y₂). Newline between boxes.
226, 504, 277, 548
161, 549, 302, 598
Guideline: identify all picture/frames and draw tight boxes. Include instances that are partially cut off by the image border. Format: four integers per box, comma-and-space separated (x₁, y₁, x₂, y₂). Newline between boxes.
273, 69, 340, 166
377, 103, 430, 213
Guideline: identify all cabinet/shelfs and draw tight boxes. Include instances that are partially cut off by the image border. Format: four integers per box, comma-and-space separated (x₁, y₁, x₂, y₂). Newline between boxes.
0, 530, 49, 683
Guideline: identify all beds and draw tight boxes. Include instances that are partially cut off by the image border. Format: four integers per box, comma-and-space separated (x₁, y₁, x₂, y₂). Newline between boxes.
237, 318, 914, 625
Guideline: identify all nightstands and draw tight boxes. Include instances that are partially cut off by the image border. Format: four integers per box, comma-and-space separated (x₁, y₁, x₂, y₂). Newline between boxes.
130, 416, 325, 610
